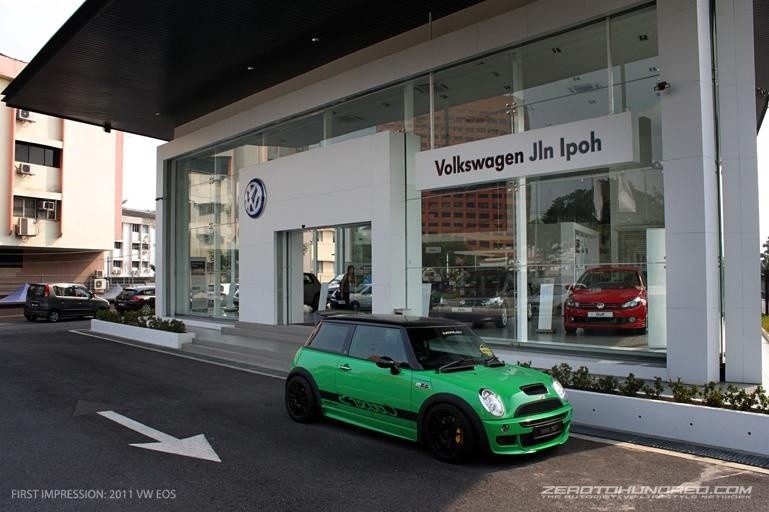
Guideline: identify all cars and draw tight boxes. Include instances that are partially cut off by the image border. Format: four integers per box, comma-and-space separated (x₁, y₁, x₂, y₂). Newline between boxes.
439, 266, 534, 327
24, 282, 110, 323
284, 311, 574, 464
233, 272, 321, 310
564, 266, 647, 333
114, 286, 155, 316
330, 284, 372, 309
328, 273, 363, 292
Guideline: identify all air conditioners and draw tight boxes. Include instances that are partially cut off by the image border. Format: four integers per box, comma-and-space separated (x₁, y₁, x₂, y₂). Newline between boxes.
92, 270, 106, 290
16, 200, 54, 236
19, 162, 33, 175
17, 109, 36, 123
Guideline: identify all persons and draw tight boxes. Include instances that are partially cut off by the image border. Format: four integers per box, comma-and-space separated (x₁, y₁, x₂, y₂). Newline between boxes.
339, 266, 355, 306
